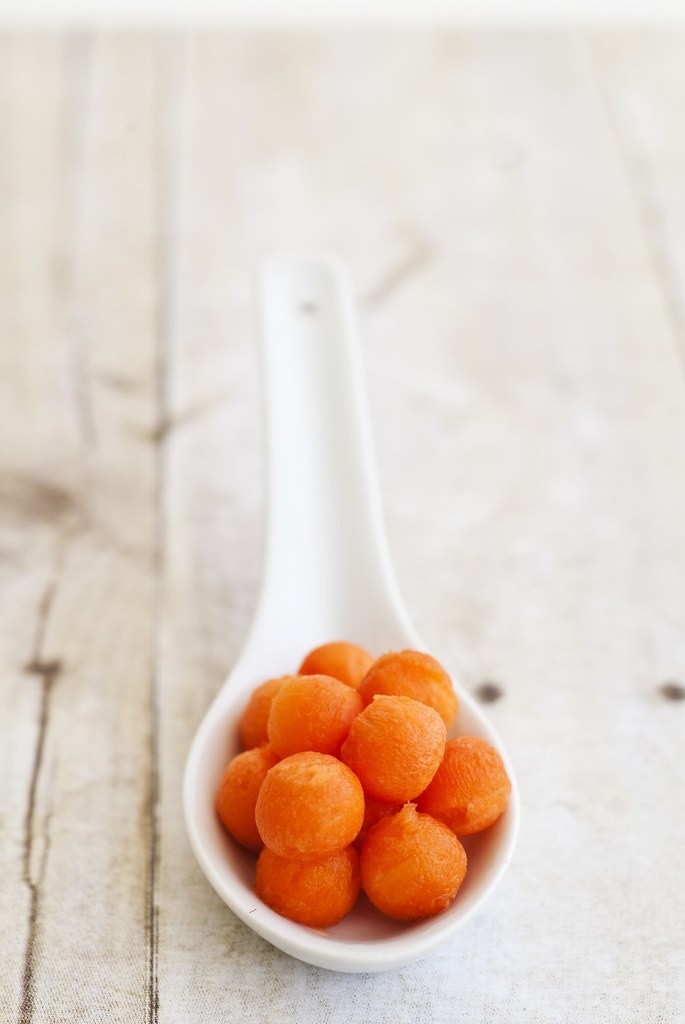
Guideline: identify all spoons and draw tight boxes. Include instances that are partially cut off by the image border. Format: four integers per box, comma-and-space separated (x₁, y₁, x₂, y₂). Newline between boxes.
181, 252, 519, 975
